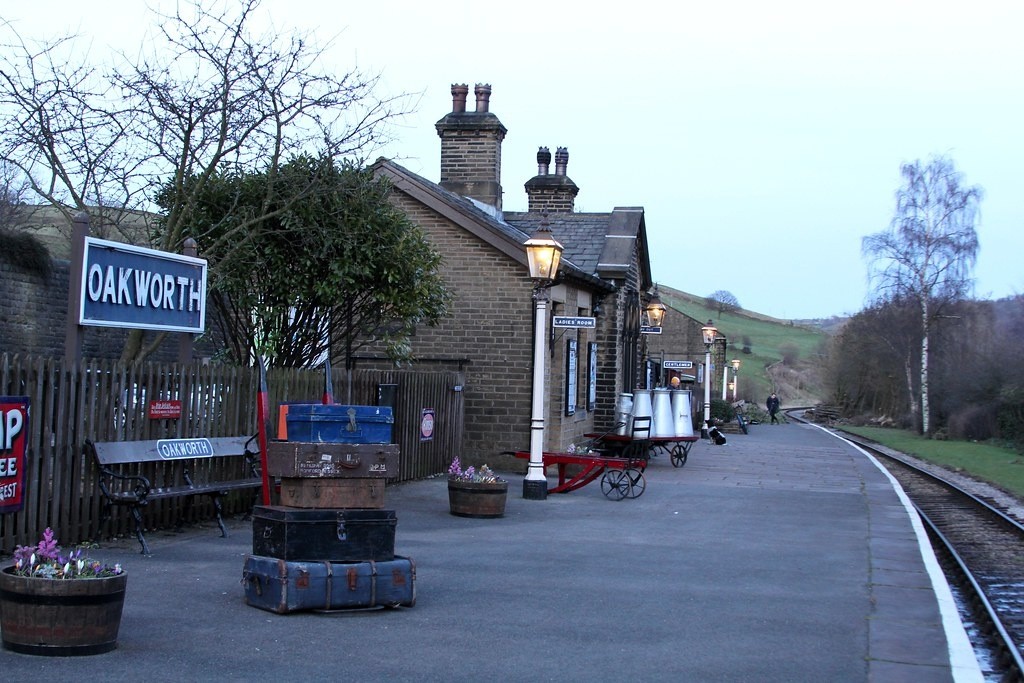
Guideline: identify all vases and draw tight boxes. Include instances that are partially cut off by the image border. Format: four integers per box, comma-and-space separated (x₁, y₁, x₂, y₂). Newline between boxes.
0, 565, 128, 657
447, 480, 508, 518
557, 453, 601, 480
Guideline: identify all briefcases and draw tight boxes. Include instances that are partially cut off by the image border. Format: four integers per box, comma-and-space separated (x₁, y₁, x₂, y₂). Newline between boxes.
281, 477, 385, 509
286, 405, 394, 444
252, 505, 398, 562
268, 442, 400, 479
242, 555, 417, 613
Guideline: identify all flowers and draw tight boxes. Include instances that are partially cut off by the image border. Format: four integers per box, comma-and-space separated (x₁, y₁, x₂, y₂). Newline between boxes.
564, 444, 593, 454
13, 527, 121, 577
448, 456, 499, 484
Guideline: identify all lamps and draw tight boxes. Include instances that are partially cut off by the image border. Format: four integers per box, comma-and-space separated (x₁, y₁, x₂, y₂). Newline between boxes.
641, 282, 667, 328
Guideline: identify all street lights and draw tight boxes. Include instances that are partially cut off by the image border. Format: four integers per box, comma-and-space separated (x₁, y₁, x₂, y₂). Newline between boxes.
701, 317, 719, 439
522, 202, 565, 497
732, 355, 741, 400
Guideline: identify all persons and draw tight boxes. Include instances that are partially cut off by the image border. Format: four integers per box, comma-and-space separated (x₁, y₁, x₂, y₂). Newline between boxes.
765, 392, 780, 425
666, 376, 680, 390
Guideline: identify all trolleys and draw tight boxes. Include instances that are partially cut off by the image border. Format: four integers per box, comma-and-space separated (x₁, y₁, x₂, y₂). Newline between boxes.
499, 450, 648, 503
584, 433, 700, 469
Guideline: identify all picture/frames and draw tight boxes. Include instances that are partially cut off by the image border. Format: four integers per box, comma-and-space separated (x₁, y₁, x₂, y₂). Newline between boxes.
586, 342, 597, 412
565, 339, 577, 416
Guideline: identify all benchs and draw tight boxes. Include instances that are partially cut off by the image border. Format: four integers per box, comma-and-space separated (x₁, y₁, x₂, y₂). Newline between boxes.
85, 433, 261, 554
